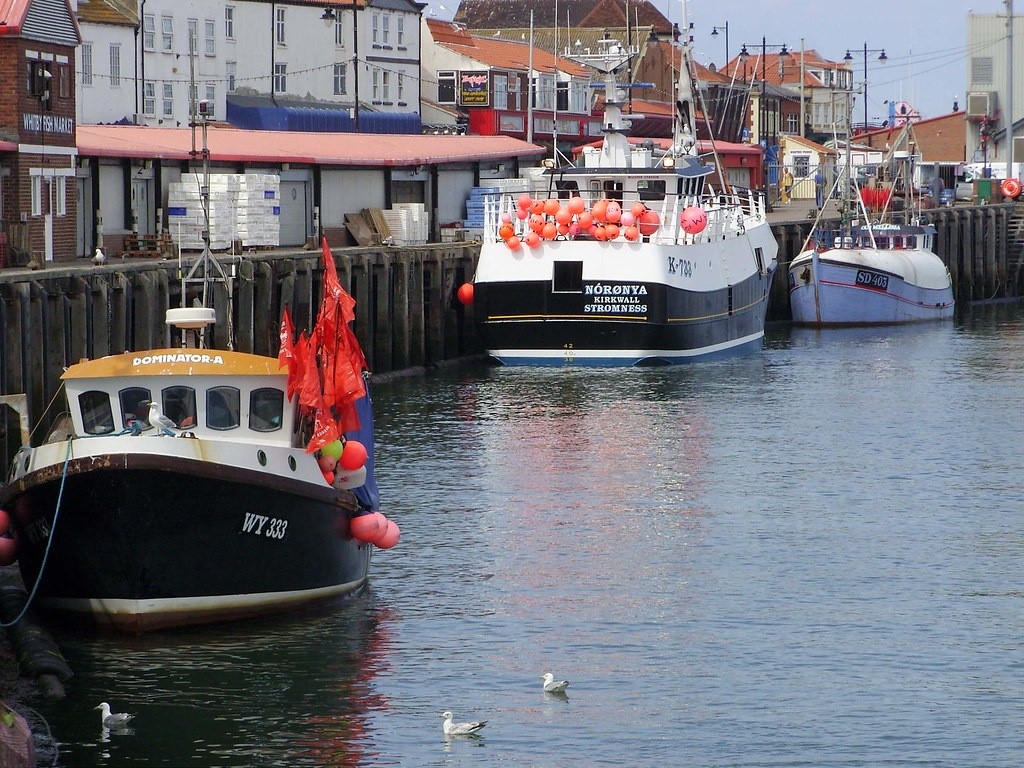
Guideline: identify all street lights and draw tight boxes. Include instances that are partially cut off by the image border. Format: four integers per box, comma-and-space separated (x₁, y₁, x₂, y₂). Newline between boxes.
842, 40, 890, 135
319, 0, 366, 133
739, 35, 789, 149
711, 20, 731, 77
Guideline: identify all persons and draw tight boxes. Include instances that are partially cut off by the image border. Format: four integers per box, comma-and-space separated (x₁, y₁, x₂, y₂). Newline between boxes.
784, 167, 793, 205
814, 170, 827, 211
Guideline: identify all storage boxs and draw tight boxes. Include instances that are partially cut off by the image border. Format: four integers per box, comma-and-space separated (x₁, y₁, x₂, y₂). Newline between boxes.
166, 171, 280, 252
441, 177, 530, 244
381, 201, 430, 247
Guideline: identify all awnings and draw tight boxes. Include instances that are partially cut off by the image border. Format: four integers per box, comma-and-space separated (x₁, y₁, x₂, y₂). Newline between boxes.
226, 95, 422, 135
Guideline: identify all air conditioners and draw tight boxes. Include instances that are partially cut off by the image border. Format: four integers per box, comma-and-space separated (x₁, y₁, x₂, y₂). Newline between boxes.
966, 90, 998, 119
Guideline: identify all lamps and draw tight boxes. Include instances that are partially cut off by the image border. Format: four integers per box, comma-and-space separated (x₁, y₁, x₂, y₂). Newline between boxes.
430, 124, 467, 136
575, 37, 582, 48
494, 163, 506, 175
411, 165, 424, 177
278, 163, 290, 175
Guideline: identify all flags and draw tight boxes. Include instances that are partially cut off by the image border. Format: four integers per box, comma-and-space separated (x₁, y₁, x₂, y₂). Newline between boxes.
277, 238, 368, 454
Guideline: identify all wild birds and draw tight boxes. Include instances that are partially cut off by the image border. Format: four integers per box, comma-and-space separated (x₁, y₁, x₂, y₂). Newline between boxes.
540, 673, 569, 692
93, 702, 136, 727
95, 248, 106, 266
438, 711, 488, 735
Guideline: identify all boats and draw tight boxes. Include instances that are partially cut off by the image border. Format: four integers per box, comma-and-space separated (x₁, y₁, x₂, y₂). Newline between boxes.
787, 114, 958, 324
10, 306, 402, 643
471, 0, 779, 371
